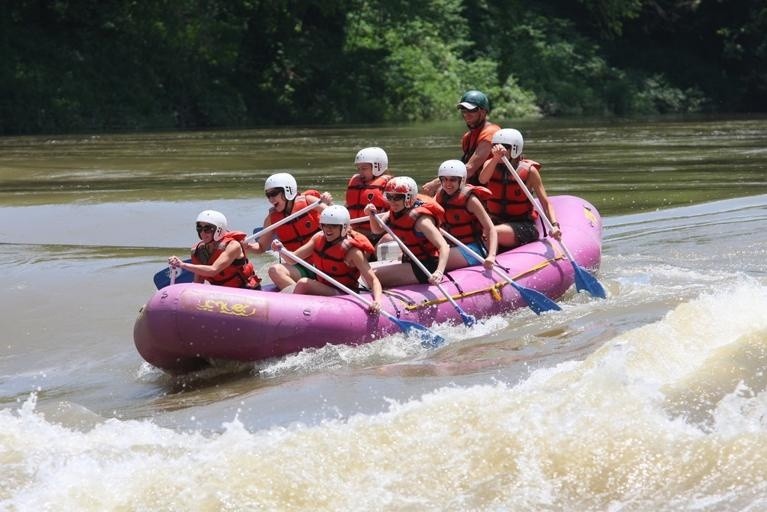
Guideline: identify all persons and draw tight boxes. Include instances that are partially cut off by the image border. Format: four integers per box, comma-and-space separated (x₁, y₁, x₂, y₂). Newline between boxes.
428, 159, 499, 272
483, 128, 562, 253
356, 176, 450, 289
240, 173, 333, 290
169, 210, 262, 291
268, 205, 382, 316
341, 147, 395, 263
417, 90, 500, 197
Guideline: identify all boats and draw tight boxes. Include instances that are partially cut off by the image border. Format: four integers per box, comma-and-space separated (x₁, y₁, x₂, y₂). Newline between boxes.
134, 191, 605, 377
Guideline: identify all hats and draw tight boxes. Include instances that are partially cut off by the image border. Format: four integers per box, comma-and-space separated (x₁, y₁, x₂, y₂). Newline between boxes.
457, 91, 489, 112
456, 101, 476, 111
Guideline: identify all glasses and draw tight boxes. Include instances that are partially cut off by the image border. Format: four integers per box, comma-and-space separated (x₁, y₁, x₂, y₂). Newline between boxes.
385, 195, 402, 200
265, 191, 280, 198
197, 226, 212, 232
440, 177, 457, 183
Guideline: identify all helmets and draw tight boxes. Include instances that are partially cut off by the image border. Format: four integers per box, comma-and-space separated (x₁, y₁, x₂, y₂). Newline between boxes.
492, 128, 523, 159
385, 176, 418, 208
318, 205, 350, 237
265, 173, 297, 201
438, 160, 467, 190
195, 210, 226, 242
354, 147, 388, 176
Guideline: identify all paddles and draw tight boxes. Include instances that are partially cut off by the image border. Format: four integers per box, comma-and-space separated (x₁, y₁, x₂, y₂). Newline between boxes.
371, 211, 477, 326
254, 211, 391, 243
502, 153, 606, 303
279, 247, 445, 348
153, 200, 323, 290
438, 225, 560, 317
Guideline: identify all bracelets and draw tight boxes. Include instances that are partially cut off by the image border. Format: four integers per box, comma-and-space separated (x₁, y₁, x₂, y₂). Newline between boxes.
552, 222, 560, 228
